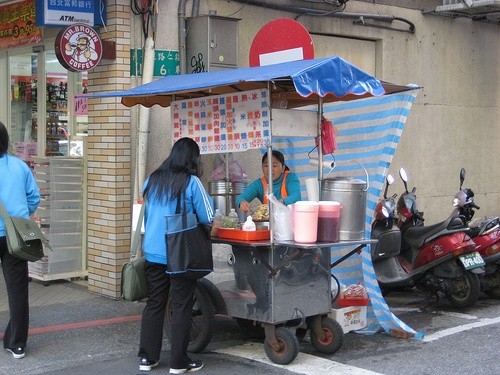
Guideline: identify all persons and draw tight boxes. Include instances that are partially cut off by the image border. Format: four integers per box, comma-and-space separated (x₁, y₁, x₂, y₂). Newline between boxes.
235, 150, 303, 328
232, 246, 273, 313
138, 137, 216, 374
0, 121, 41, 359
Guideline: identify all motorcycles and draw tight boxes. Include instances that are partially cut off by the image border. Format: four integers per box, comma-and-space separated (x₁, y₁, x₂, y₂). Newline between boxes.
370, 166, 500, 312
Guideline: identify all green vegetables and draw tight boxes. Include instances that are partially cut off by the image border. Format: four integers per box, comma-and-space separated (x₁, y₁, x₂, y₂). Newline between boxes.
222, 217, 237, 229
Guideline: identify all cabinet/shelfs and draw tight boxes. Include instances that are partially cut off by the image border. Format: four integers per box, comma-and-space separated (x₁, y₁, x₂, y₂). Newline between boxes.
32, 76, 88, 156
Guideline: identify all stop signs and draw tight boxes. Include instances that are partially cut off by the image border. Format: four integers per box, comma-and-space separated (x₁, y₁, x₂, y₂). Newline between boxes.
248, 17, 315, 69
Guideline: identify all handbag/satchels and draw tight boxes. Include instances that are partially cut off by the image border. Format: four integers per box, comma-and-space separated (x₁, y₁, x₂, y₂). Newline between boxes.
210, 159, 248, 182
165, 186, 213, 280
4, 216, 49, 262
121, 256, 148, 302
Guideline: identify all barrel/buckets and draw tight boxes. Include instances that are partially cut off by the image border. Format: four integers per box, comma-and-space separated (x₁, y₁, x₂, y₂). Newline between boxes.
321, 160, 369, 240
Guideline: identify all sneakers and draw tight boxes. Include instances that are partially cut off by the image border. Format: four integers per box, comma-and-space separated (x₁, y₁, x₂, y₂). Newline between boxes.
169, 360, 204, 374
7, 346, 25, 358
139, 357, 159, 371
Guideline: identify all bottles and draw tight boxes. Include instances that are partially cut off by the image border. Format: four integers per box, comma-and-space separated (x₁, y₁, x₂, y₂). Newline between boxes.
46, 123, 51, 135
317, 201, 343, 243
46, 82, 59, 102
340, 286, 346, 299
64, 123, 67, 135
242, 215, 256, 231
51, 123, 56, 135
59, 82, 64, 100
294, 201, 318, 243
64, 83, 68, 101
57, 123, 62, 134
214, 209, 223, 227
229, 208, 238, 219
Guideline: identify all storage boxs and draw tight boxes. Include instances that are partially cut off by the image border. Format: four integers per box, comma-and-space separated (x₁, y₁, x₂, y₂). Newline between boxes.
338, 299, 368, 306
328, 306, 367, 335
214, 228, 270, 240
